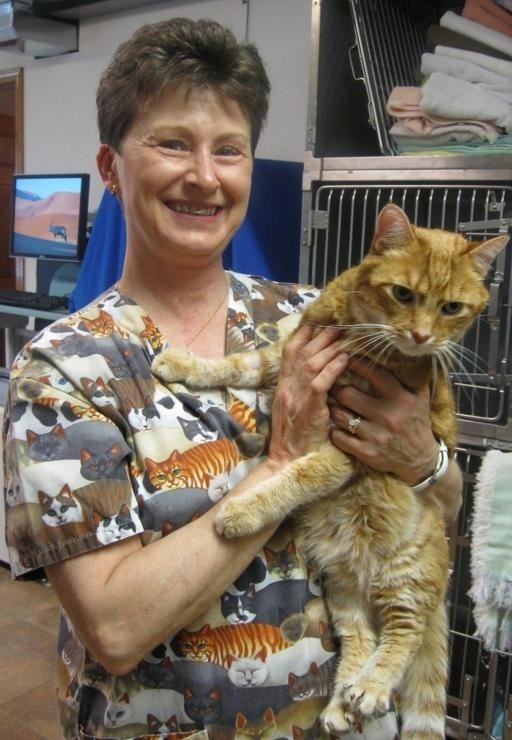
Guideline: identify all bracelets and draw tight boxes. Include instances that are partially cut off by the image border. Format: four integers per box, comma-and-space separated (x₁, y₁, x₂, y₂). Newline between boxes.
407, 433, 449, 494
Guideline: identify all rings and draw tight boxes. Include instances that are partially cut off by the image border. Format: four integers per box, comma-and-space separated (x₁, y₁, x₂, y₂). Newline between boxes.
347, 416, 361, 437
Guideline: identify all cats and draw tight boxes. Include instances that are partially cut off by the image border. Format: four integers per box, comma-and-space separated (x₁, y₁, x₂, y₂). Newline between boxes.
149, 200, 510, 740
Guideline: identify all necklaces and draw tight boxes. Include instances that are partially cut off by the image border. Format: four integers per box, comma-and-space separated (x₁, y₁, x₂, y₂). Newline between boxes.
175, 283, 236, 359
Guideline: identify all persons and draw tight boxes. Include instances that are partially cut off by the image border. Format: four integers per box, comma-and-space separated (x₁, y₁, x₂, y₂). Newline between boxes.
6, 16, 460, 739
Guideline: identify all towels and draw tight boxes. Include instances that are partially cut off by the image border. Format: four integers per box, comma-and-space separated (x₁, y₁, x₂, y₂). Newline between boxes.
379, 2, 512, 158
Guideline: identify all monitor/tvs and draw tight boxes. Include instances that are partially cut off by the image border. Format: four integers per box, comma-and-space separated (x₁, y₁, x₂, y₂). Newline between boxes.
8, 173, 89, 262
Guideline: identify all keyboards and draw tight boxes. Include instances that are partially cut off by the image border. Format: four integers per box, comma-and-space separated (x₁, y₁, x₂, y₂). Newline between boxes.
0, 289, 62, 311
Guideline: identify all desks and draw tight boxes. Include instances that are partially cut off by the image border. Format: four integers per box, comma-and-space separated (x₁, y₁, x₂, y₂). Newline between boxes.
0, 304, 88, 584
